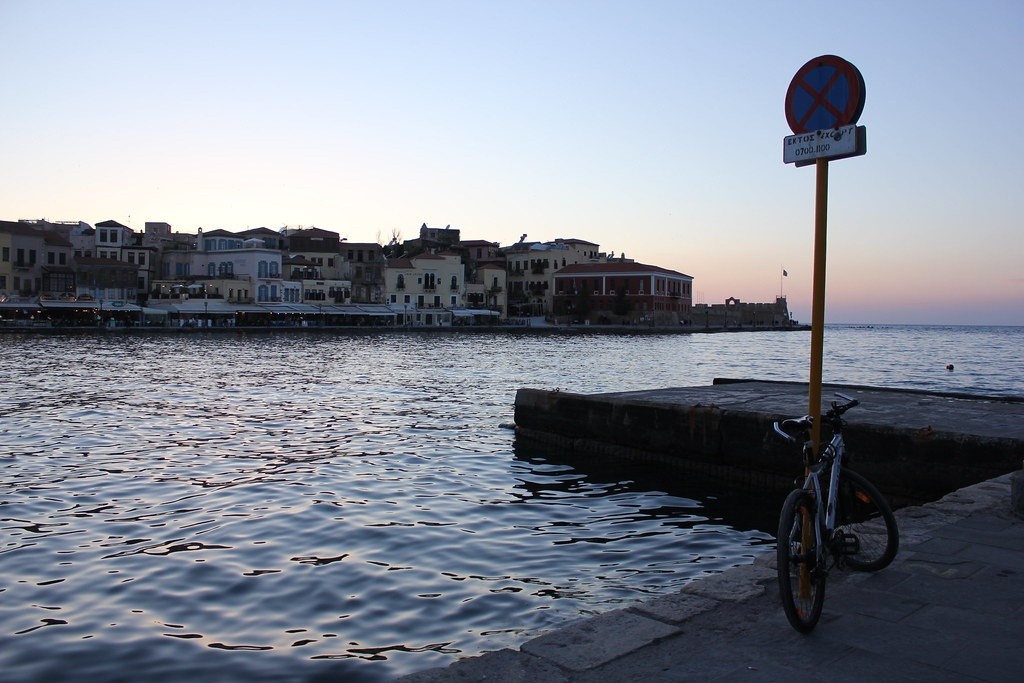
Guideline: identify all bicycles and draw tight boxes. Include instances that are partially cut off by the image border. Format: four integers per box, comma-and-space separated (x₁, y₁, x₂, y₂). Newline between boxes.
771, 391, 901, 636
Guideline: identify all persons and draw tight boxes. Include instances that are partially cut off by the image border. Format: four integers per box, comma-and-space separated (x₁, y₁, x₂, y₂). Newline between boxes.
439, 318, 442, 326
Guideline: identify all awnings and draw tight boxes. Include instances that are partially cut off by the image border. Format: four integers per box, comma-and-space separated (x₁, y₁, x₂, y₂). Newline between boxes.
171, 284, 203, 299
141, 302, 416, 325
450, 309, 500, 325
0, 298, 142, 323
